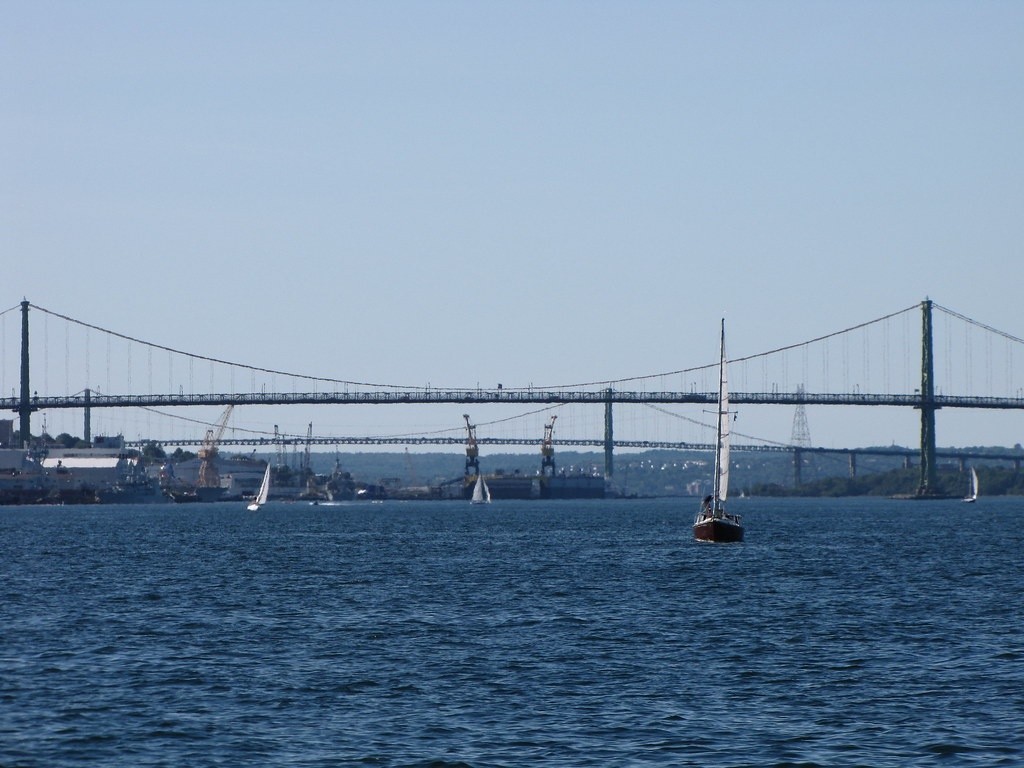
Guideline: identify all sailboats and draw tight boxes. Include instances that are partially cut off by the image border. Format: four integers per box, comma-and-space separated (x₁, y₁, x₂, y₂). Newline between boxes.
961, 465, 978, 503
693, 318, 745, 544
471, 473, 491, 504
247, 458, 271, 512
